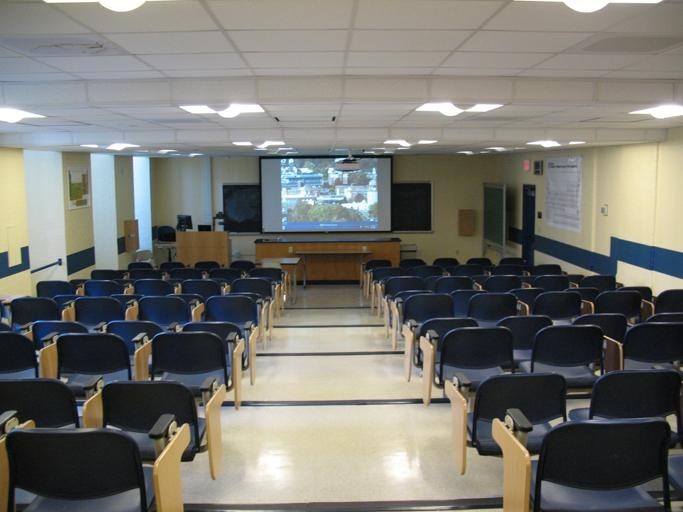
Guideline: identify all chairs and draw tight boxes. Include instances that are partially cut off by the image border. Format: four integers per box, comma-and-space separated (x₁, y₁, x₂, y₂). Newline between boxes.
150, 331, 247, 410
498, 258, 525, 265
666, 455, 683, 491
138, 296, 192, 325
75, 296, 124, 323
84, 280, 124, 297
529, 275, 570, 291
8, 422, 192, 512
160, 262, 185, 271
70, 279, 90, 286
182, 279, 222, 298
452, 263, 485, 276
0, 378, 80, 429
106, 321, 165, 356
654, 289, 683, 314
52, 295, 84, 308
169, 268, 203, 280
111, 294, 145, 305
194, 261, 220, 269
400, 258, 427, 268
531, 290, 582, 318
482, 274, 522, 293
128, 262, 153, 270
10, 298, 60, 326
568, 369, 683, 450
32, 321, 89, 351
531, 264, 562, 274
36, 280, 73, 298
573, 313, 626, 344
568, 273, 584, 284
383, 290, 433, 337
496, 315, 553, 351
56, 333, 133, 394
248, 268, 287, 319
579, 276, 615, 290
166, 280, 184, 286
466, 257, 492, 266
492, 414, 671, 512
510, 287, 546, 307
409, 266, 443, 277
492, 264, 522, 276
90, 270, 125, 280
451, 290, 488, 317
0, 331, 39, 378
363, 267, 405, 298
433, 258, 460, 267
435, 276, 474, 294
565, 288, 601, 301
519, 325, 604, 396
418, 327, 515, 409
183, 322, 259, 386
373, 276, 424, 318
263, 257, 301, 304
230, 278, 278, 339
622, 322, 683, 371
444, 371, 566, 476
390, 294, 456, 349
102, 382, 226, 480
647, 311, 683, 322
359, 260, 392, 288
209, 268, 242, 282
205, 296, 269, 351
129, 270, 162, 279
133, 279, 175, 297
468, 291, 517, 322
230, 260, 256, 272
115, 280, 136, 286
402, 317, 479, 383
616, 286, 652, 303
594, 290, 641, 318
226, 292, 260, 302
168, 294, 205, 307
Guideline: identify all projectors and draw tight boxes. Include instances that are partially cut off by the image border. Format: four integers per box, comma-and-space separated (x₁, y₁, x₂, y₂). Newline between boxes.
334, 159, 360, 172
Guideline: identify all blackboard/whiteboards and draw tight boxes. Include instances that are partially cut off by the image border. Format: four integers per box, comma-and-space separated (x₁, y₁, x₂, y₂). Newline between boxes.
482, 181, 506, 249
221, 180, 434, 234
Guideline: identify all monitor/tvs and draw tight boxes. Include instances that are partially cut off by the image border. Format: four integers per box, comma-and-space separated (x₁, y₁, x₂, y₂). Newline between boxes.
177, 214, 193, 232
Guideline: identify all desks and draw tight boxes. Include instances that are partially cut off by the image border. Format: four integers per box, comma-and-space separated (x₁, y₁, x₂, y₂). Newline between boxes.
295, 249, 374, 289
254, 238, 401, 285
176, 231, 232, 267
